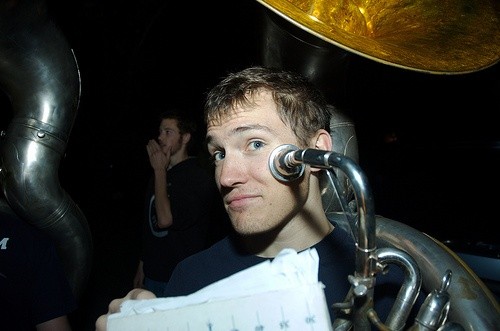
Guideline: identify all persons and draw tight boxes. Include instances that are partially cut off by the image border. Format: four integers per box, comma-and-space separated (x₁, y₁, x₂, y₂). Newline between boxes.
94, 66, 456, 331
132, 114, 216, 290
0, 194, 71, 331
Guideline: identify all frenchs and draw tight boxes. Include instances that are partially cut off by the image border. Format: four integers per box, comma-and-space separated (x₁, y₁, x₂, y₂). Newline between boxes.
256, 0, 498, 331
